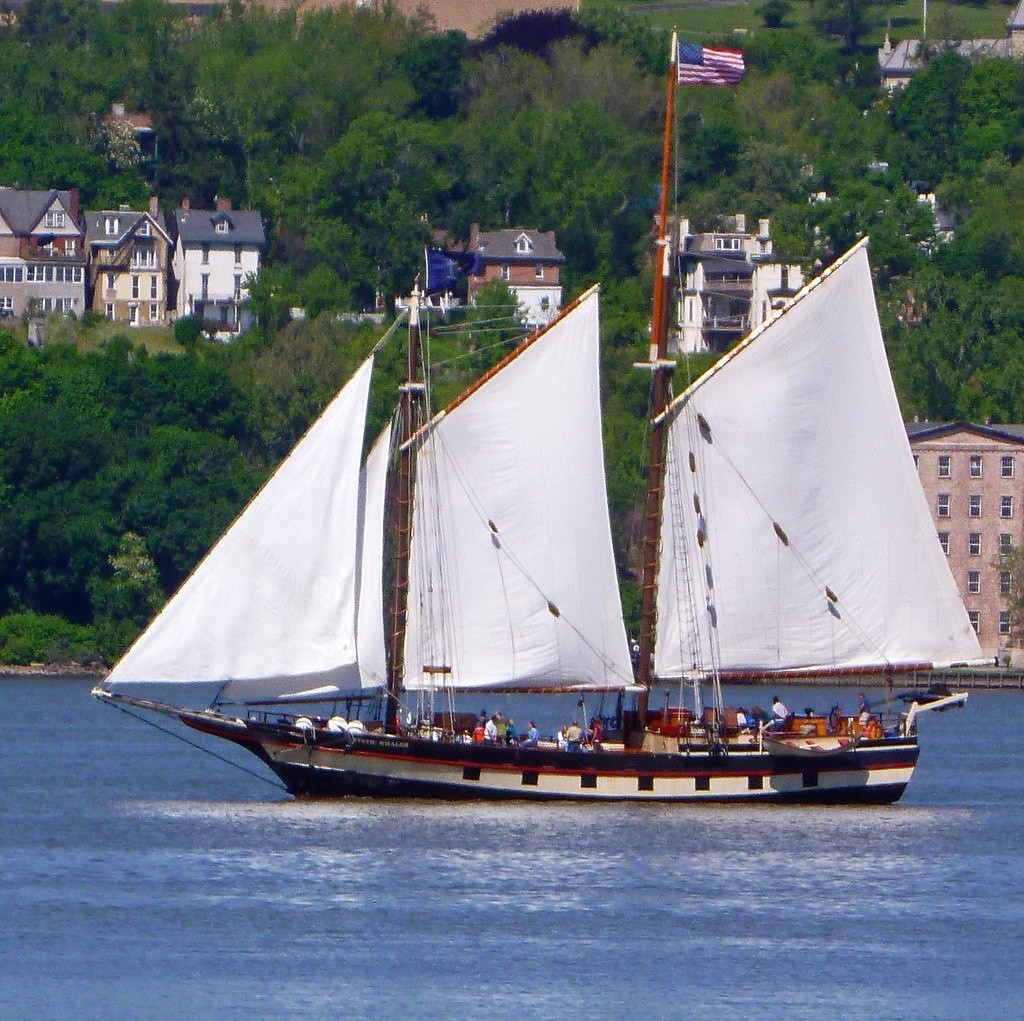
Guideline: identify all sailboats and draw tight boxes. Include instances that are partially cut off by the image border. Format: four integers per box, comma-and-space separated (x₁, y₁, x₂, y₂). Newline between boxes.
91, 24, 998, 806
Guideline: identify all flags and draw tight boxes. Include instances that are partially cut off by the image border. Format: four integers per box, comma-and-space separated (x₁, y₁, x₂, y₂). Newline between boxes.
425, 248, 486, 293
676, 41, 744, 86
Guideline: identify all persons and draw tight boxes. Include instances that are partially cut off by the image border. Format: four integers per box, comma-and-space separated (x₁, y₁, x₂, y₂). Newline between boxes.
857, 693, 871, 728
471, 708, 607, 754
736, 696, 788, 735
